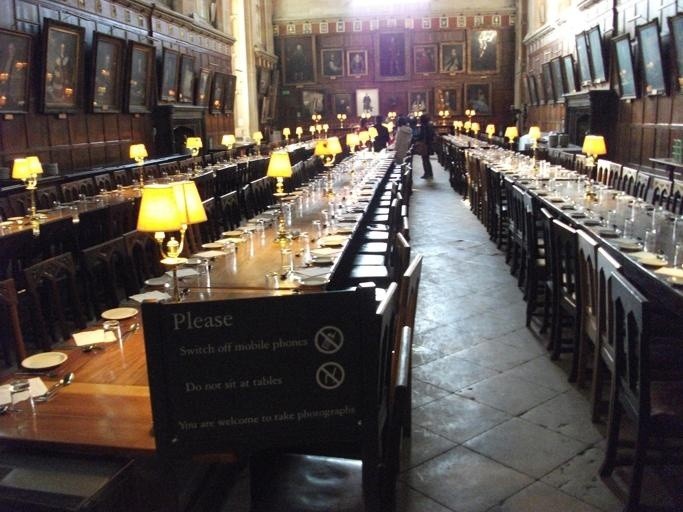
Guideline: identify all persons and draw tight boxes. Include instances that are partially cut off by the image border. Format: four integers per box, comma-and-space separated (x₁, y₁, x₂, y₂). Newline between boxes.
354, 114, 438, 180
274, 32, 495, 116
1, 40, 233, 110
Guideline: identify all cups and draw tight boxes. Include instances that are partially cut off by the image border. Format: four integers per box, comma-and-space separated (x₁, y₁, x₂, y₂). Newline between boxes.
9, 379, 41, 421
102, 319, 124, 353
264, 198, 336, 288
1, 151, 267, 227
197, 257, 209, 277
489, 142, 683, 265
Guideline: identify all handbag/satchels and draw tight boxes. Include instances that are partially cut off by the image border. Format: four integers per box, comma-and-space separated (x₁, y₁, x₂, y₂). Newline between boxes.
414, 142, 428, 155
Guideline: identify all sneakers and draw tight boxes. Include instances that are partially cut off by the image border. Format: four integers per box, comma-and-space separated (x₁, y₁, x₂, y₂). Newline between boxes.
421, 173, 433, 179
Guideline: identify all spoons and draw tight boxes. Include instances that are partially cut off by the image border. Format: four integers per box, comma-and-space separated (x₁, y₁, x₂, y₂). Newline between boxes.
121, 323, 140, 344
59, 342, 95, 355
178, 286, 192, 302
35, 372, 74, 405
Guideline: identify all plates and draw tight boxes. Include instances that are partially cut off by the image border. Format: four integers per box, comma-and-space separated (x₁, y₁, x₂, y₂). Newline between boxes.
6, 215, 25, 221
19, 350, 69, 370
101, 307, 140, 321
37, 209, 49, 213
296, 205, 365, 289
143, 278, 171, 286
197, 172, 329, 251
1, 221, 13, 226
